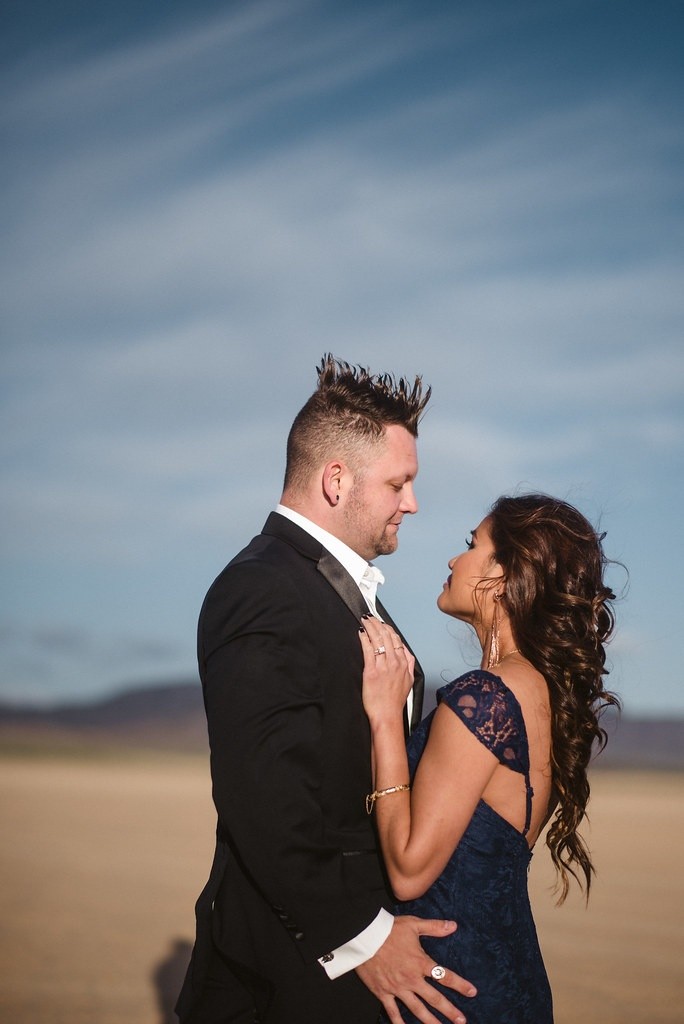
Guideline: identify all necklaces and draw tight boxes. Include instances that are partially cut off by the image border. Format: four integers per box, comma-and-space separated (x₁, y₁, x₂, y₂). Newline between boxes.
486, 648, 522, 671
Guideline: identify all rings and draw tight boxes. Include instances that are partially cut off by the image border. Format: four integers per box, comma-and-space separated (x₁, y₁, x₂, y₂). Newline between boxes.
393, 646, 404, 649
374, 646, 386, 656
431, 966, 447, 980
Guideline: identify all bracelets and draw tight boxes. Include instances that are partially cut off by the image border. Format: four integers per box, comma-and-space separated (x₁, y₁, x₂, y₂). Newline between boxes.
367, 784, 411, 811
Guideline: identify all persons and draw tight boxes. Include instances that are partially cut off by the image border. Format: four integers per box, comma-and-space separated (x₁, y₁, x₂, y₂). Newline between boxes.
357, 493, 622, 1024
175, 349, 480, 1024
